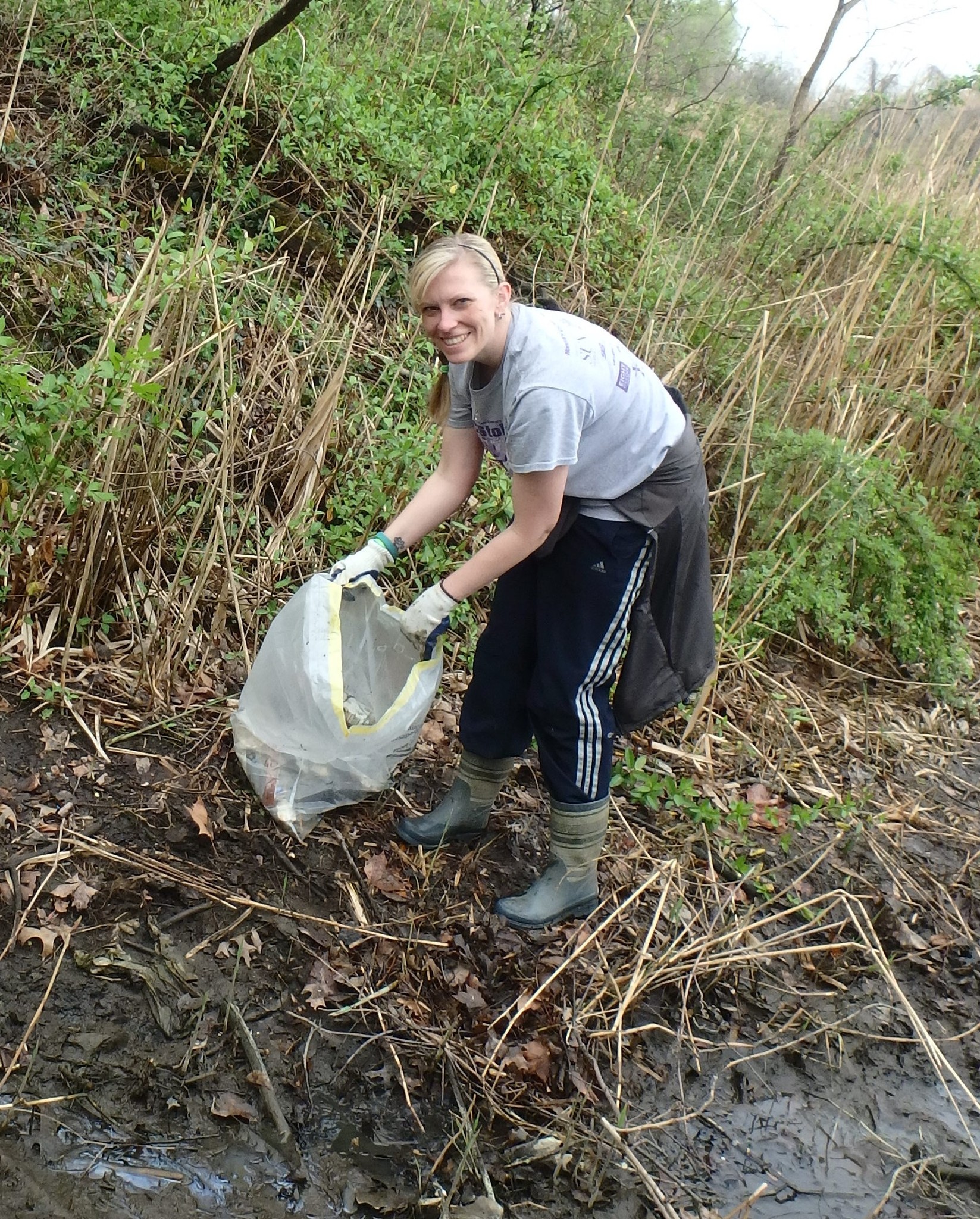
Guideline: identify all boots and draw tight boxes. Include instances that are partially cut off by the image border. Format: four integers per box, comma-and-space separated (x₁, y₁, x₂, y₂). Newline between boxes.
494, 790, 611, 932
393, 750, 515, 849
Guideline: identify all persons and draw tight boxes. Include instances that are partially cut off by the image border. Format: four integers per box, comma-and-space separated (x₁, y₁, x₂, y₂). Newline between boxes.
327, 232, 718, 931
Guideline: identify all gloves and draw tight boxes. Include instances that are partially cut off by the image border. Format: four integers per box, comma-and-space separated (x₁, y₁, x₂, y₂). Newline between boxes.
327, 531, 399, 602
400, 578, 462, 662
